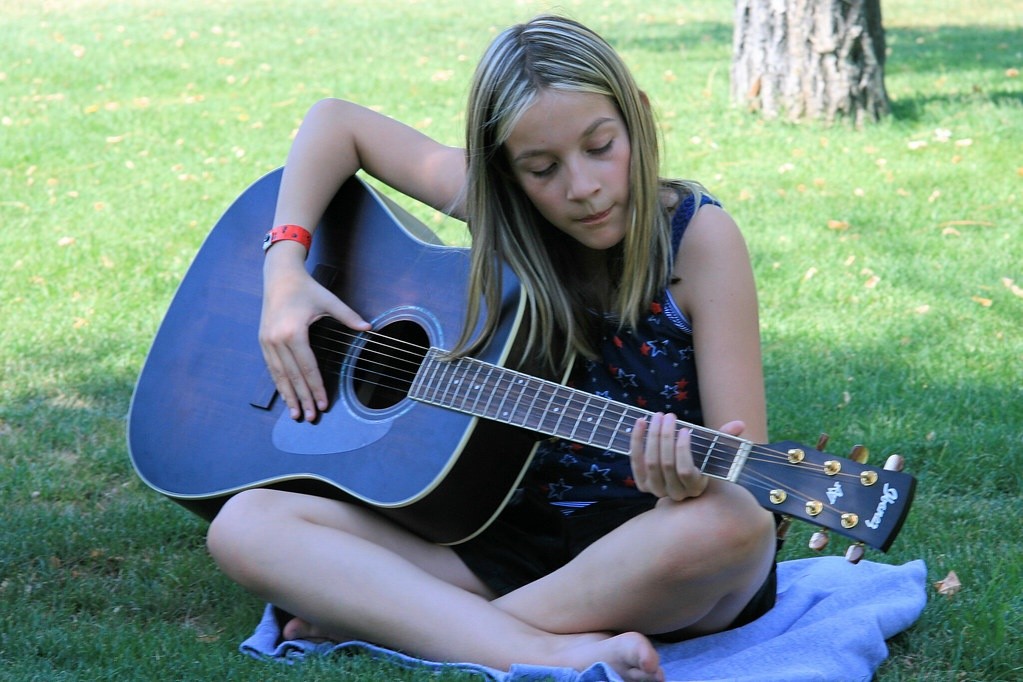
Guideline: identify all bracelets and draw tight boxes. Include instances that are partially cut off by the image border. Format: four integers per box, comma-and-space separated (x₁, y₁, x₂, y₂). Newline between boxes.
262, 224, 312, 261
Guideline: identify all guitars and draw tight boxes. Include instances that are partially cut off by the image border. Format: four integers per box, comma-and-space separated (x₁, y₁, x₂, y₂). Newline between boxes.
118, 154, 921, 570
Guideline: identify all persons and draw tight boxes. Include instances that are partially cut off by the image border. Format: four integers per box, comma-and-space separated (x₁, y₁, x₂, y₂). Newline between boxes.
206, 15, 777, 682
